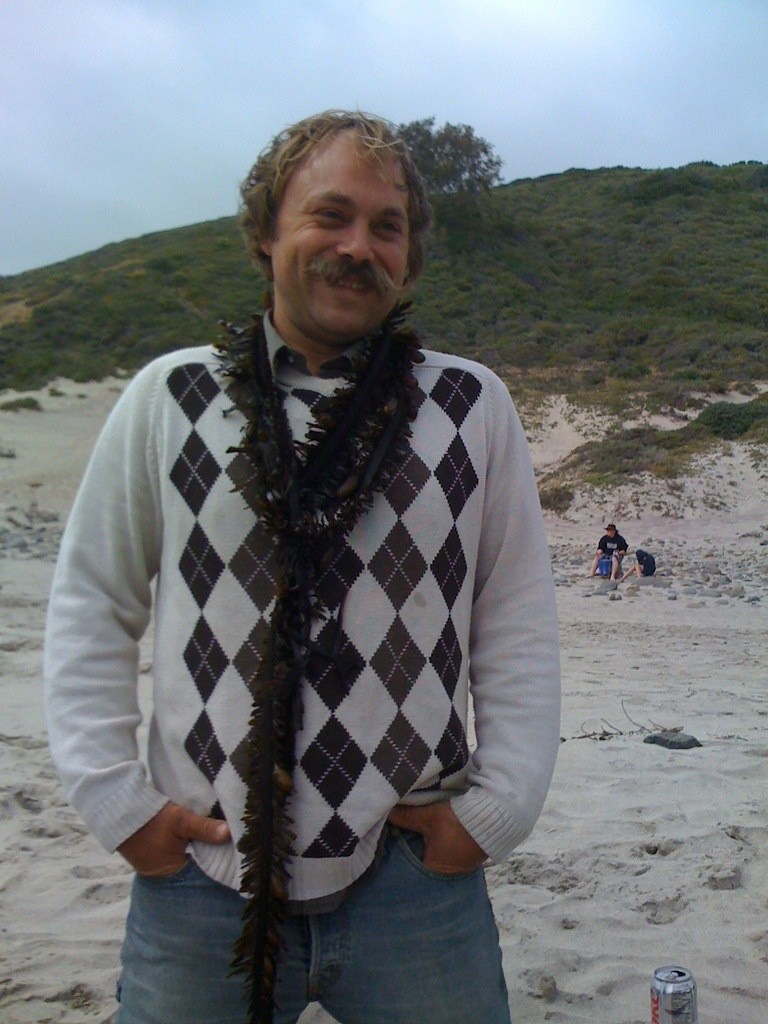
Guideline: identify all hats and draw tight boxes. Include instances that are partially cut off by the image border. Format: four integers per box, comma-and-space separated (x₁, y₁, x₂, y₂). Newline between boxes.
604, 524, 617, 530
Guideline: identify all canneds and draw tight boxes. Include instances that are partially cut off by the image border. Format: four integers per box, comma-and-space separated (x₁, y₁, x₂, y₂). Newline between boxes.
649, 966, 699, 1023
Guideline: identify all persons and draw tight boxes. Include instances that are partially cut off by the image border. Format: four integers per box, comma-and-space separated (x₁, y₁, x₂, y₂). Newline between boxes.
619, 549, 656, 583
589, 523, 628, 580
43, 109, 561, 1024
